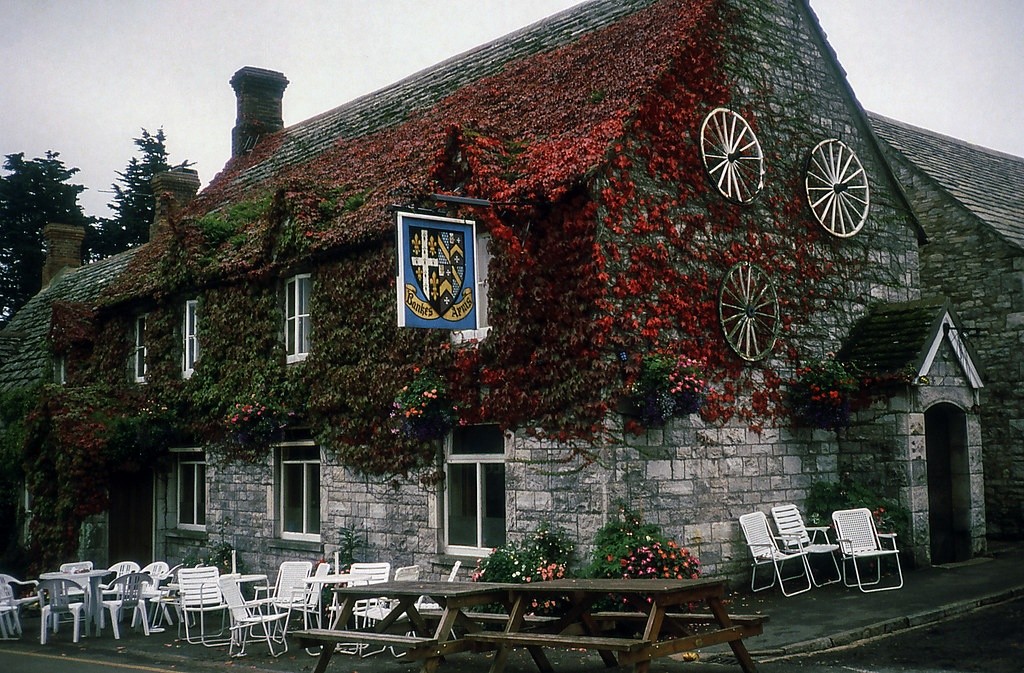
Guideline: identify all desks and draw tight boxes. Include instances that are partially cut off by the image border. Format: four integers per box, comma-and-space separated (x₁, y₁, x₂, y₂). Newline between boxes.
39, 570, 111, 632
303, 574, 371, 656
489, 579, 758, 673
312, 579, 522, 673
200, 575, 270, 647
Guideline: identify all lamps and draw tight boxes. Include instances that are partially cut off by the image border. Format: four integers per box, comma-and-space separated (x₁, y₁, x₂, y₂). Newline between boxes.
943, 322, 989, 338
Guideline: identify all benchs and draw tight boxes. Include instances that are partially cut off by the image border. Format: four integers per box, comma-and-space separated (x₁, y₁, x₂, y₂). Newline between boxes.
291, 612, 771, 673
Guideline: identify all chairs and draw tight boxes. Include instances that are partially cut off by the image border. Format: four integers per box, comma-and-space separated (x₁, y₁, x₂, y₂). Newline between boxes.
771, 504, 842, 588
739, 511, 811, 597
0, 560, 462, 658
832, 508, 904, 593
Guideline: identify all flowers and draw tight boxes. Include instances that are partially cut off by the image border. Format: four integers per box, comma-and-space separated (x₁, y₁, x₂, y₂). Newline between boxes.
470, 498, 702, 608
